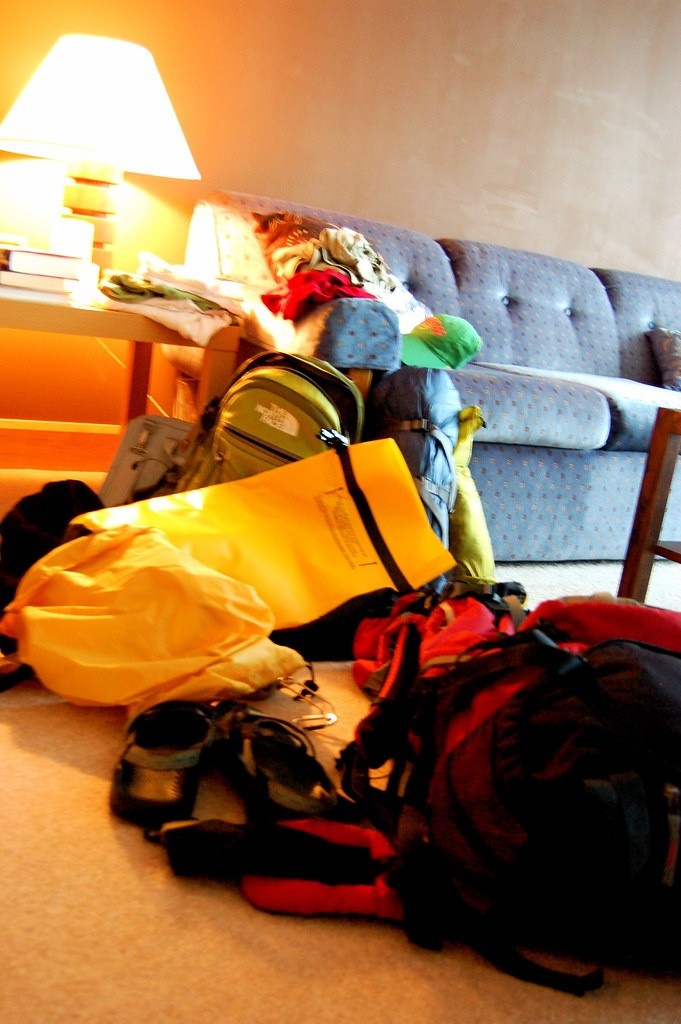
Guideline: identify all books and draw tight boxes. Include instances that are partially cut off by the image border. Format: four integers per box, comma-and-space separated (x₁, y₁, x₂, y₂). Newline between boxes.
0, 267, 84, 304
0, 248, 80, 281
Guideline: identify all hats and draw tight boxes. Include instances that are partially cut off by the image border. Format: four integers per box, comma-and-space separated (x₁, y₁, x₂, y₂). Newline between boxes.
316, 295, 405, 377
398, 313, 483, 370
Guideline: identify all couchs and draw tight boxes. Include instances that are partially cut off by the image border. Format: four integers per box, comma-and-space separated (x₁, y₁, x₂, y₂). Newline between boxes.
306, 208, 681, 564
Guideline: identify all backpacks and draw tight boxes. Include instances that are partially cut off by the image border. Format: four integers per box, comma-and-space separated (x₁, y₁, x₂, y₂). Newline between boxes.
357, 364, 434, 479
164, 351, 365, 498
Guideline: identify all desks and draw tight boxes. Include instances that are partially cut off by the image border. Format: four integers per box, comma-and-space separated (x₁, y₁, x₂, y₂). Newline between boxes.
0, 271, 244, 420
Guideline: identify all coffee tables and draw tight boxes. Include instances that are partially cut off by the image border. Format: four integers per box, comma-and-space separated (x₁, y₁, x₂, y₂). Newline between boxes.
617, 407, 681, 603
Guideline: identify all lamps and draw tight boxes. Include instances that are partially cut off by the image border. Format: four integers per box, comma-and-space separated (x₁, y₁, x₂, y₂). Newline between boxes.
0, 34, 200, 282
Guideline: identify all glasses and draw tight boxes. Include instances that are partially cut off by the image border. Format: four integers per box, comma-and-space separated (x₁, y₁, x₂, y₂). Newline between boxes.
127, 699, 313, 784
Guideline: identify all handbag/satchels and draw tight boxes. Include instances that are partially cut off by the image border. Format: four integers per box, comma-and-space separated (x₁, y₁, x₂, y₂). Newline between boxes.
159, 596, 681, 998
97, 408, 199, 518
0, 526, 312, 712
